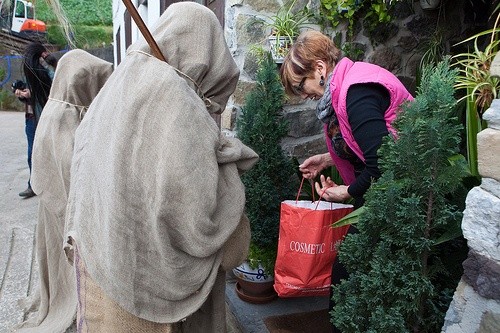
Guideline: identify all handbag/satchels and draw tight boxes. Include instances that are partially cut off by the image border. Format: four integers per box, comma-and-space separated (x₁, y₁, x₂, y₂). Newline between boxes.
273, 172, 355, 298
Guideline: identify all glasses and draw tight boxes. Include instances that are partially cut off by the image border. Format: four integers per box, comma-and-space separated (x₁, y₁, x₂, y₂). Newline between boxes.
294, 77, 307, 95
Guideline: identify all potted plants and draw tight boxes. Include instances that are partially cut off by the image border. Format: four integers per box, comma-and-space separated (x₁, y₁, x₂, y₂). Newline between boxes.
232, 50, 300, 304
245, 0, 317, 63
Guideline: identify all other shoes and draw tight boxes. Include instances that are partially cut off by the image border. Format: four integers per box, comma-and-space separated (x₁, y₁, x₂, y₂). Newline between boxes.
19, 188, 35, 197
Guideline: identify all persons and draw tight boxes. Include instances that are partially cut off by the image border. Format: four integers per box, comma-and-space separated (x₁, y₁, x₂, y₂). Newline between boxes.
10, 1, 260, 333
280, 28, 417, 309
